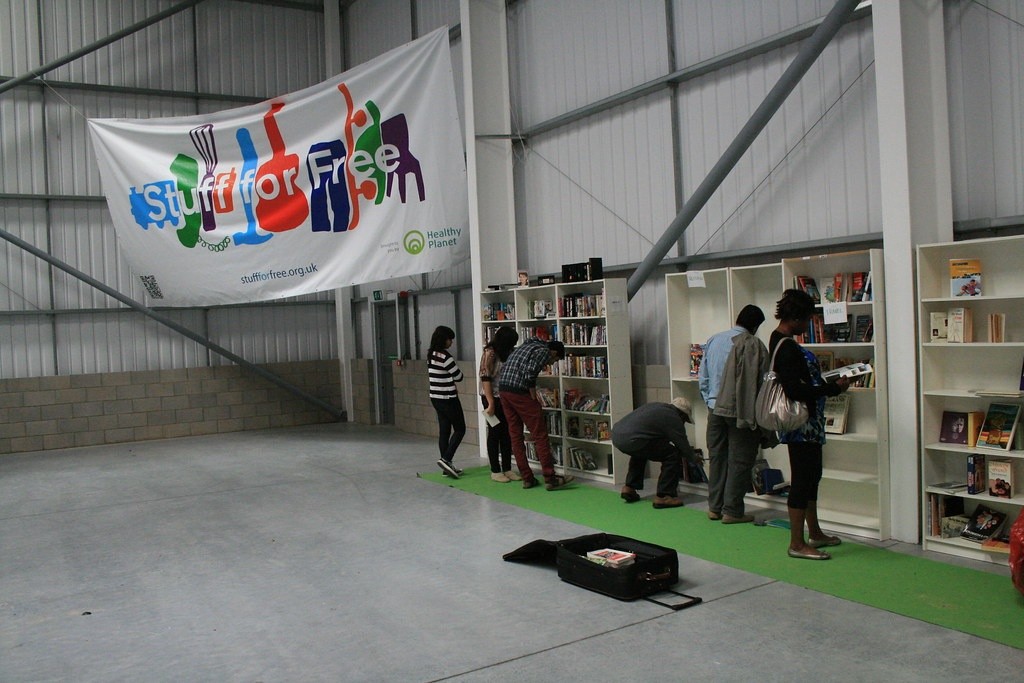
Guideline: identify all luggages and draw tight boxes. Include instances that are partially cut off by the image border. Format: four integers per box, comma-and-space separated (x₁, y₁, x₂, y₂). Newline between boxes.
502, 532, 704, 612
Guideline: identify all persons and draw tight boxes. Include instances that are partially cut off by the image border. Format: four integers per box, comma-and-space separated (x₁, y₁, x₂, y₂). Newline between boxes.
478, 326, 523, 483
426, 325, 466, 478
960, 280, 978, 296
518, 273, 528, 287
769, 289, 850, 560
989, 479, 1012, 498
698, 304, 772, 524
943, 417, 965, 443
499, 337, 574, 491
612, 397, 703, 509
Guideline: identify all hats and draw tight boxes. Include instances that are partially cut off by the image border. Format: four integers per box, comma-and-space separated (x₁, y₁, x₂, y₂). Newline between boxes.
671, 397, 695, 425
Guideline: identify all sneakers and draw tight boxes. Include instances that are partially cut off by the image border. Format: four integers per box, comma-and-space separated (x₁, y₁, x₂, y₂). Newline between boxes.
437, 459, 463, 479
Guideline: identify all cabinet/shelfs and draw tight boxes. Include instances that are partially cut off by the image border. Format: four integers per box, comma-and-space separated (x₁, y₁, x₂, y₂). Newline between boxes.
915, 236, 1024, 566
665, 249, 892, 542
480, 279, 632, 487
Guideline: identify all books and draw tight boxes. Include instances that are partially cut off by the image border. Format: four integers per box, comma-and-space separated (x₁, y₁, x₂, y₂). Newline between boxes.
930, 307, 1006, 344
586, 548, 637, 568
950, 258, 982, 297
675, 343, 791, 499
927, 493, 1008, 545
481, 257, 613, 476
988, 459, 1017, 500
791, 270, 877, 435
940, 402, 1024, 451
928, 454, 985, 494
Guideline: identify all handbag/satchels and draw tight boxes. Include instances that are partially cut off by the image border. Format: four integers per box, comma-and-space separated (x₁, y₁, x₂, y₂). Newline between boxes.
755, 337, 809, 431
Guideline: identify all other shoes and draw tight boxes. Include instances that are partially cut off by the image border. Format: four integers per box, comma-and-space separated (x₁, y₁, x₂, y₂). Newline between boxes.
545, 474, 574, 490
491, 470, 522, 482
808, 536, 842, 548
653, 494, 684, 508
620, 486, 640, 503
787, 548, 830, 559
708, 511, 754, 524
522, 477, 539, 489
